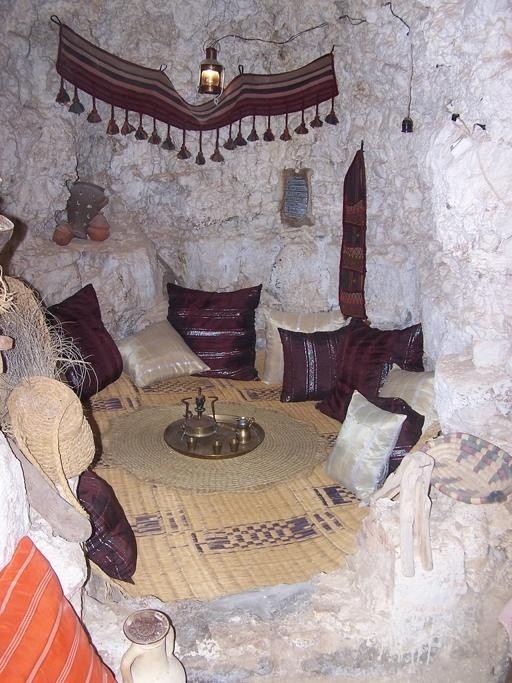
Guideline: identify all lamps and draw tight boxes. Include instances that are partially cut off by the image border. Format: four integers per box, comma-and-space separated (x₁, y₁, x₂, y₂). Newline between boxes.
196, 42, 225, 96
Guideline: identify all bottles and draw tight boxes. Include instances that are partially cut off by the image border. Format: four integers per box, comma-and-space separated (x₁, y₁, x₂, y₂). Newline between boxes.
118, 610, 187, 683
86, 210, 110, 241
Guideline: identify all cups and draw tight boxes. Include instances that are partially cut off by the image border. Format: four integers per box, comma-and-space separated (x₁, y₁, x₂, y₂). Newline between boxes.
229, 438, 239, 451
212, 440, 223, 454
187, 436, 197, 449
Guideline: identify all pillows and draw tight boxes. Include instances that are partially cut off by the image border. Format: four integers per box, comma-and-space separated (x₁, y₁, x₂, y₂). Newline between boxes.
0, 534, 119, 683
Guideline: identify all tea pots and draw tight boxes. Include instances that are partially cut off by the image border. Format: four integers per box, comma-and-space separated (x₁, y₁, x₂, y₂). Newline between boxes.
232, 416, 255, 444
